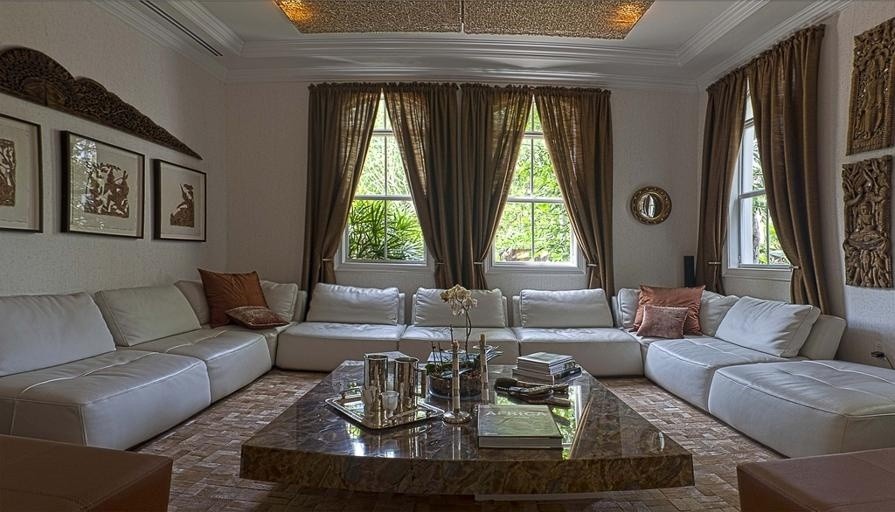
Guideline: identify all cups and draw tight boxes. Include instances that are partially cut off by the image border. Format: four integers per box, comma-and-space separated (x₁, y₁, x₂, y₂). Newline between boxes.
360, 354, 389, 408
380, 390, 399, 411
393, 356, 425, 411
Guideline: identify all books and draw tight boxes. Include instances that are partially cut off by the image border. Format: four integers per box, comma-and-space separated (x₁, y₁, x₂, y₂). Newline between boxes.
476, 404, 565, 451
363, 350, 412, 375
510, 351, 583, 382
426, 350, 469, 376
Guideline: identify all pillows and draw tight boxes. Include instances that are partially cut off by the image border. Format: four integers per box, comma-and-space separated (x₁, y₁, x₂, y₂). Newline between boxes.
628, 283, 708, 339
195, 266, 289, 331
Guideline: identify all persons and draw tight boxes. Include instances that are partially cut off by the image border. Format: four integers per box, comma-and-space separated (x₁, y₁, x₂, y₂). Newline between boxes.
863, 58, 884, 141
849, 198, 880, 242
845, 177, 885, 213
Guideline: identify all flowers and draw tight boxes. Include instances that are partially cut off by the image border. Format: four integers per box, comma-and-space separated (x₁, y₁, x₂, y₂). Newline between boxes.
437, 287, 478, 361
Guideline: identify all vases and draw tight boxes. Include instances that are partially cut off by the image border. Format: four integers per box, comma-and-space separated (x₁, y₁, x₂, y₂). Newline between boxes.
427, 354, 484, 400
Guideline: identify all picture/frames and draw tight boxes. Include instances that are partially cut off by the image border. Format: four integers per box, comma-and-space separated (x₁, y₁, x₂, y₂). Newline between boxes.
1, 110, 207, 240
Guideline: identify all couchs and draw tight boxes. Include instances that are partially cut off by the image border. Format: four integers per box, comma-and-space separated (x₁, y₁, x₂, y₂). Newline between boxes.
0, 278, 306, 450
274, 284, 642, 376
610, 271, 892, 457
